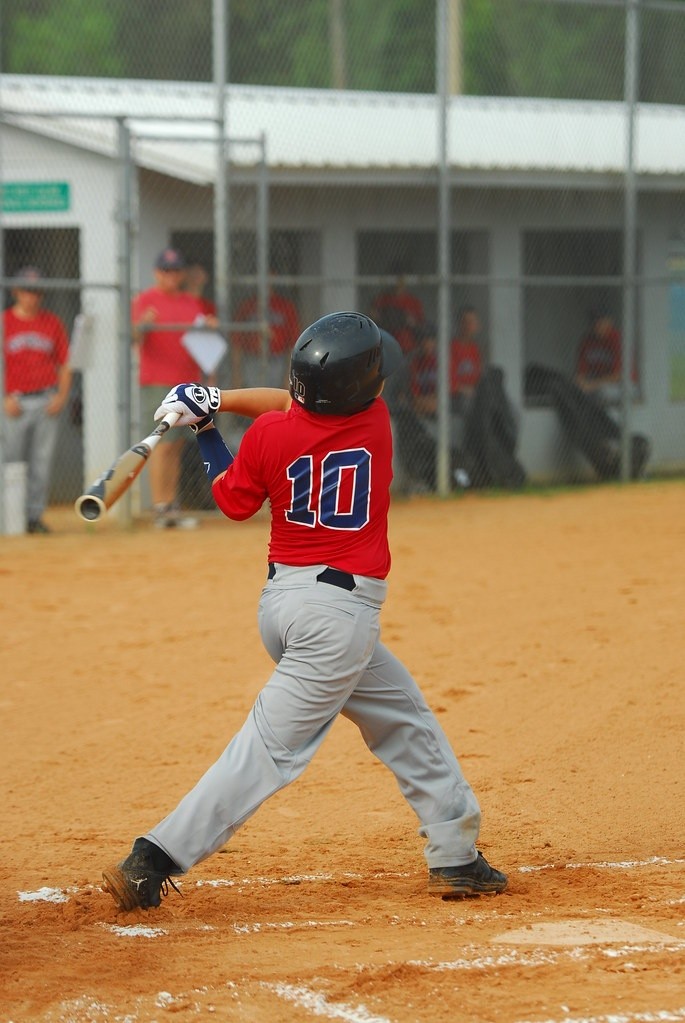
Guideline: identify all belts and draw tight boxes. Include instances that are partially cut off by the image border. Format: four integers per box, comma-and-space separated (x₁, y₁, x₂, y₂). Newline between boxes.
266, 564, 387, 604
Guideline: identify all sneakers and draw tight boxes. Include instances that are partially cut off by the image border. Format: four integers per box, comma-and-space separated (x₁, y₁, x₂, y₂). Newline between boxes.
103, 842, 165, 911
429, 853, 511, 898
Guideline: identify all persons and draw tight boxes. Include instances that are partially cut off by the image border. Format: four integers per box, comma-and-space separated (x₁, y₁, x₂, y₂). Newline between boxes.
124, 241, 222, 535
574, 311, 639, 418
1, 261, 76, 539
364, 260, 531, 489
228, 260, 303, 433
173, 255, 225, 499
98, 311, 510, 913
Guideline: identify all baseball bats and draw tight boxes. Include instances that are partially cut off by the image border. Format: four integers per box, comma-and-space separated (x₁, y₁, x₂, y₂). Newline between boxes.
75, 412, 182, 522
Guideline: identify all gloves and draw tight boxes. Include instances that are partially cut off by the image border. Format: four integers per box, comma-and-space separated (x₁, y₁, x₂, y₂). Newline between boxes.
154, 383, 222, 433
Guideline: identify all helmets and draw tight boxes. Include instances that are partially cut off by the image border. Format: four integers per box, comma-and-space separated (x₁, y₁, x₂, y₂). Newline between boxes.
287, 311, 401, 415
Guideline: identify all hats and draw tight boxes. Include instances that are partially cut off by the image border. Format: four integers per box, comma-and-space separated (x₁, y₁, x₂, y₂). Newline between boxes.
13, 267, 48, 297
155, 248, 185, 271
